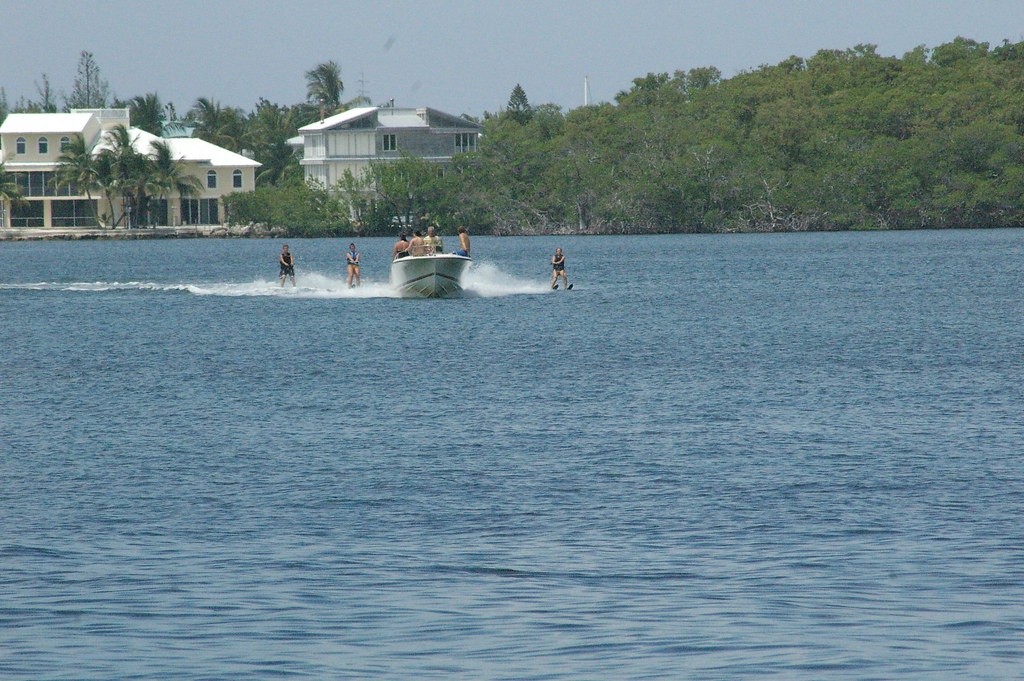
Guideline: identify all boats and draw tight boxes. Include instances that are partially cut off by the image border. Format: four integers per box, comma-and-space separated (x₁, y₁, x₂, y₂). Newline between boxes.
390, 244, 474, 297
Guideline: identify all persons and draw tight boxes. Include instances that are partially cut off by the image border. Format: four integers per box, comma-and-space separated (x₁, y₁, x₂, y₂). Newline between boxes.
392, 233, 409, 259
405, 229, 425, 257
423, 226, 443, 255
345, 243, 360, 288
452, 226, 471, 257
550, 247, 567, 289
279, 245, 296, 287
